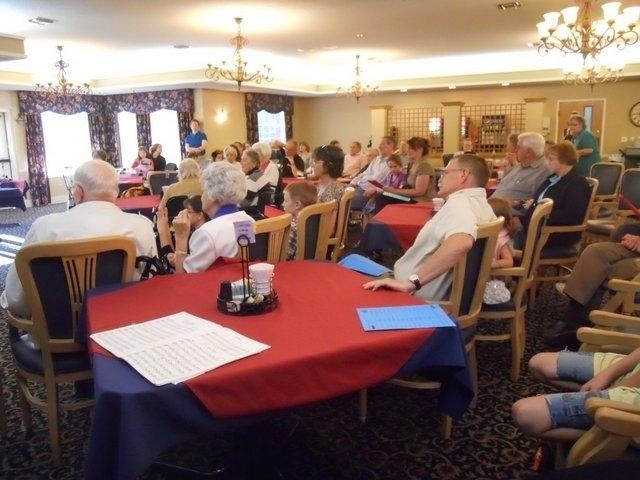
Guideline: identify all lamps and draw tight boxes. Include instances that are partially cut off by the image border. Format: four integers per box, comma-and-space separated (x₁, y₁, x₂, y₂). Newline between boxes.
533, 1, 640, 94
204, 17, 274, 92
335, 54, 379, 104
32, 46, 92, 103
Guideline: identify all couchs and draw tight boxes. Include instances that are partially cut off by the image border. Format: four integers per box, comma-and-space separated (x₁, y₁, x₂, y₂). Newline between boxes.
0, 179, 29, 212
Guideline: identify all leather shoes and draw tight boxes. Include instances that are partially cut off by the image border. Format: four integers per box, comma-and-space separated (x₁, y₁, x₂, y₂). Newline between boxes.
543, 321, 579, 337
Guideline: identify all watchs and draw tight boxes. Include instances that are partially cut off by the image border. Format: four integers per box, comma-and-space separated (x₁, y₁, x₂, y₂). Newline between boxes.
408, 274, 422, 290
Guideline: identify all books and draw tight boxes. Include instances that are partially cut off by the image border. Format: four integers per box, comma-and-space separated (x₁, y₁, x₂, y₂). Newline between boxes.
92, 310, 273, 386
354, 302, 457, 332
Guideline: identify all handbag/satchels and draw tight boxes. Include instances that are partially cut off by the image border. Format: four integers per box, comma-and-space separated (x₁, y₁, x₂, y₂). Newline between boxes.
483, 279, 511, 304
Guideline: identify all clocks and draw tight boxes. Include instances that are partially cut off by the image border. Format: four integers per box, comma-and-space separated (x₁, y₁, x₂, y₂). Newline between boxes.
629, 101, 640, 128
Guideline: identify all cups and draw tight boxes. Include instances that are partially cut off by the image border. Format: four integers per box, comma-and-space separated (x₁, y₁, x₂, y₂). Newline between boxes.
248, 262, 275, 297
432, 198, 444, 212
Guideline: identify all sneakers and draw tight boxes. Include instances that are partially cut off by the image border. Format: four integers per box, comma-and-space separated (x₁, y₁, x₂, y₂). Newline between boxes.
555, 282, 571, 300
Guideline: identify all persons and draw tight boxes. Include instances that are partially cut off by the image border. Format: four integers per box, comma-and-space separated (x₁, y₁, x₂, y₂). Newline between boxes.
91, 115, 366, 275
1, 158, 162, 319
510, 345, 640, 441
539, 235, 640, 343
346, 115, 602, 285
362, 154, 501, 297
